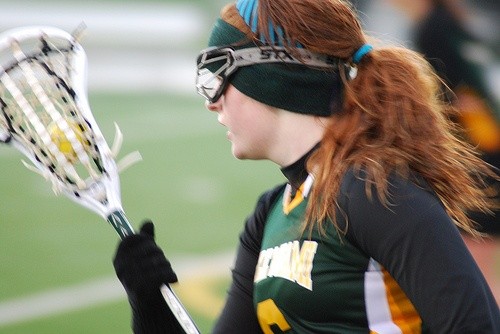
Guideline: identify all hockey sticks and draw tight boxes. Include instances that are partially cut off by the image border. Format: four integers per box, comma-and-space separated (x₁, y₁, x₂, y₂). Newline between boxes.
0, 23, 204, 333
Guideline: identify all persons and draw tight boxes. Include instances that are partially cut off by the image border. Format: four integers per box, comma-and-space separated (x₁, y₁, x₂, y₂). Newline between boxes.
392, 1, 499, 306
111, 0, 500, 334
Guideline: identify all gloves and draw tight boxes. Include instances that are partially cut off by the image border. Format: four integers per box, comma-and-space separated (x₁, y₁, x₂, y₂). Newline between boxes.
114, 218, 180, 334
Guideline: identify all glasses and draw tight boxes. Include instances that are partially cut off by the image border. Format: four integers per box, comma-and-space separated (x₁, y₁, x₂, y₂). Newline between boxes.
194, 43, 336, 104
